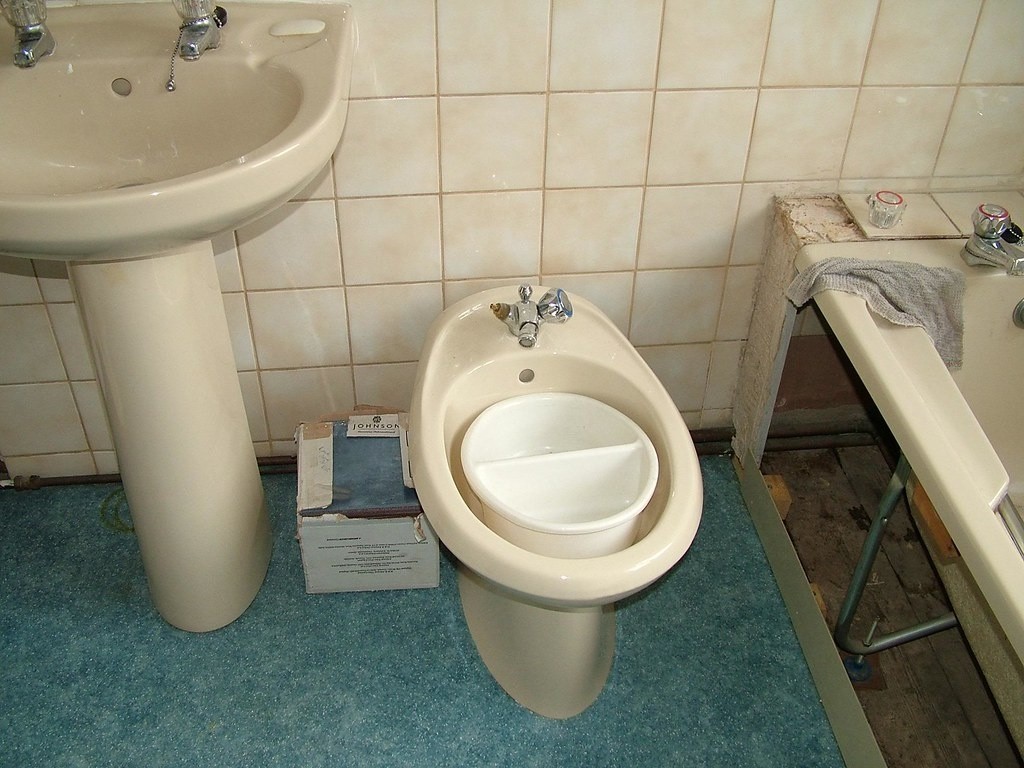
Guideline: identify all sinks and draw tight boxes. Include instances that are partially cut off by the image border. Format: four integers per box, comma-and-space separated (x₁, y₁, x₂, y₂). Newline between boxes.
0, 0, 356, 260
405, 282, 707, 723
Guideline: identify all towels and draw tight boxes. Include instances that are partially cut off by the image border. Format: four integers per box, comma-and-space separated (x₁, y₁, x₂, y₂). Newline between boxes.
783, 256, 966, 372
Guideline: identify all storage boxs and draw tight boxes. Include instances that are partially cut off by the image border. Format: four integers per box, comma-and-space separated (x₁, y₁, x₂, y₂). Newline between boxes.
293, 405, 441, 595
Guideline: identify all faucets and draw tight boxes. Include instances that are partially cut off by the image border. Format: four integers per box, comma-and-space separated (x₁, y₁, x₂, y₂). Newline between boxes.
958, 200, 1024, 278
488, 283, 574, 350
0, 1, 58, 72
166, 2, 229, 63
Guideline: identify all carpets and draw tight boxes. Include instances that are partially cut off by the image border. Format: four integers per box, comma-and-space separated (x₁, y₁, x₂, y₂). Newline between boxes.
1, 456, 846, 768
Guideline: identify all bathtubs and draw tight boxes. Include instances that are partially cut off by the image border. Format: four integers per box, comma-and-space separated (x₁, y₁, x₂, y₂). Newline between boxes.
788, 235, 1024, 667
